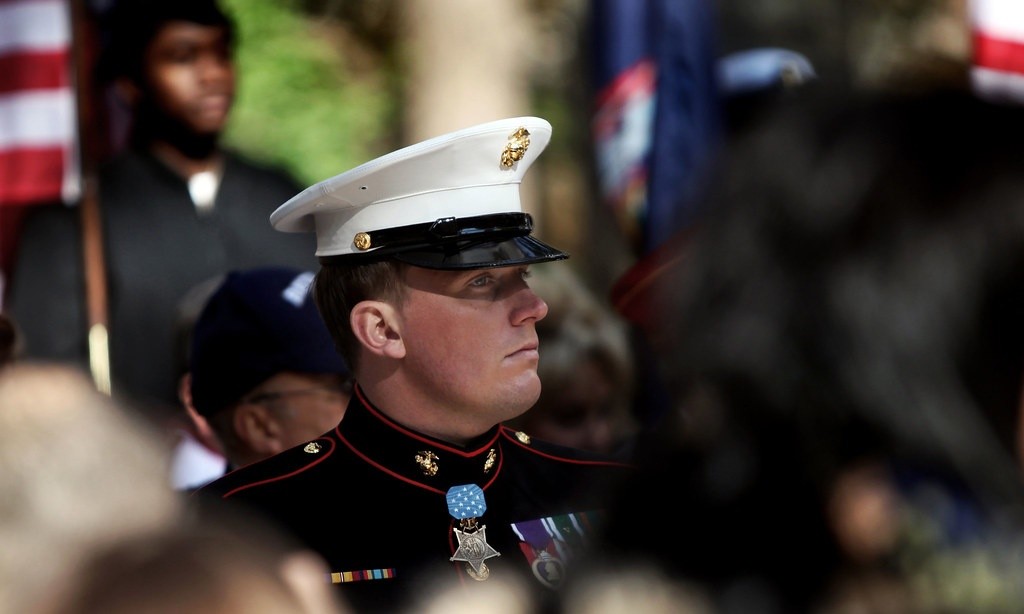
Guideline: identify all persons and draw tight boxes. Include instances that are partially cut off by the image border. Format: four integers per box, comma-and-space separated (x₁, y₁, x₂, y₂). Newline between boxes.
188, 268, 353, 479
182, 116, 653, 614
14, 0, 319, 401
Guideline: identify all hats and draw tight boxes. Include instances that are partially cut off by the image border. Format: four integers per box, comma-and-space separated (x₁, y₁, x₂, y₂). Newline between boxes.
271, 116, 569, 269
86, 0, 240, 79
189, 269, 340, 399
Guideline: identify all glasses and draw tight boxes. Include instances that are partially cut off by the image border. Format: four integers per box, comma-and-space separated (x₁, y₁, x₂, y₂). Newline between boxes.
252, 388, 349, 407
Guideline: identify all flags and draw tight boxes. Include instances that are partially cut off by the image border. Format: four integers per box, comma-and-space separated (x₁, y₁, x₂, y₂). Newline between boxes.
0, 0, 83, 204
564, 1, 709, 230
